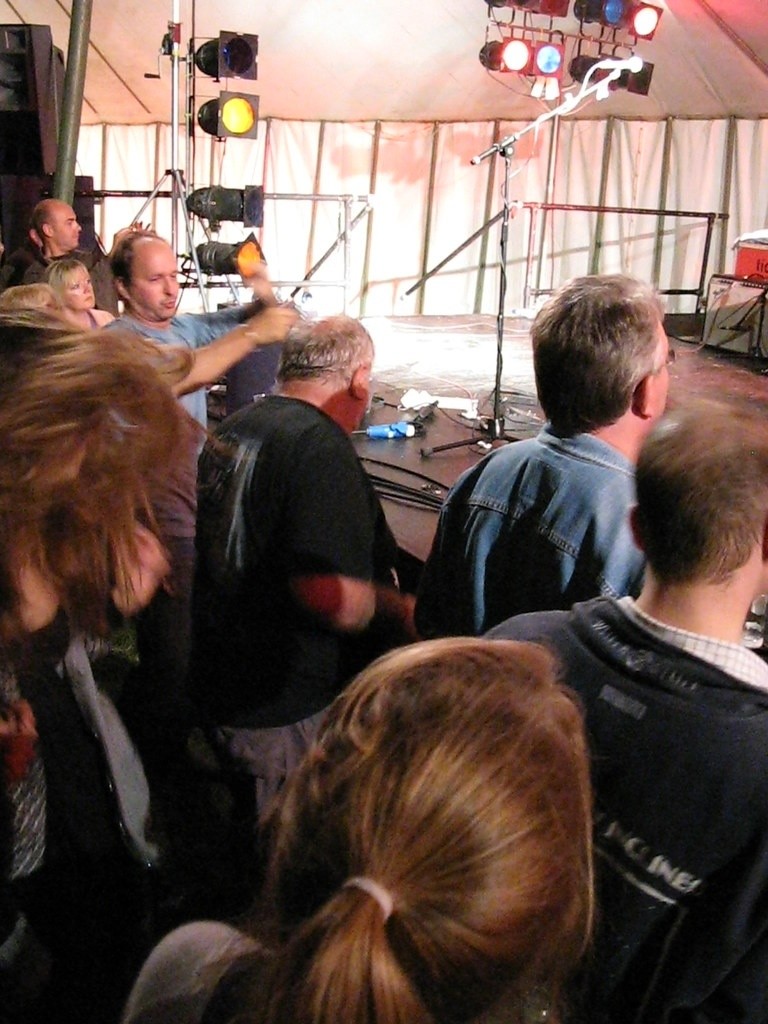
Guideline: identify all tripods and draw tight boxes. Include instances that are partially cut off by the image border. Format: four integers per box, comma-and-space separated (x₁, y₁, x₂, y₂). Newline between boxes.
420, 69, 622, 458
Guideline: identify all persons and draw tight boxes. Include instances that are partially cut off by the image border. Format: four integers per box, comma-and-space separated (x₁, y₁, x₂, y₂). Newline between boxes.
412, 275, 670, 640
0, 199, 300, 1024
183, 315, 417, 894
485, 398, 768, 1024
119, 636, 598, 1024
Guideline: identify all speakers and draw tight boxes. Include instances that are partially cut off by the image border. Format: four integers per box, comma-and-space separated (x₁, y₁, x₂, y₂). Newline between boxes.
0, 23, 95, 268
701, 275, 768, 359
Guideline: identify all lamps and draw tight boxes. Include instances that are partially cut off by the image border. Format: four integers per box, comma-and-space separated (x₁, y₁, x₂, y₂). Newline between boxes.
480, 37, 564, 78
185, 185, 265, 230
574, 0, 663, 41
485, 0, 571, 17
568, 54, 654, 95
197, 91, 259, 139
190, 231, 266, 288
193, 30, 259, 83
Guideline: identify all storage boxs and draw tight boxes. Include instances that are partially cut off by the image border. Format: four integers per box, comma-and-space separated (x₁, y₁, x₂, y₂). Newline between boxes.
701, 241, 768, 359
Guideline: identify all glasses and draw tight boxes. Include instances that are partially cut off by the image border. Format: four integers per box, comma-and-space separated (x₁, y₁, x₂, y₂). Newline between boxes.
653, 349, 676, 375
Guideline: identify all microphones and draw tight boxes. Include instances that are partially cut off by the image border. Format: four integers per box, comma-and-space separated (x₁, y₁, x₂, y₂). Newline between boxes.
597, 56, 645, 73
719, 326, 748, 332
